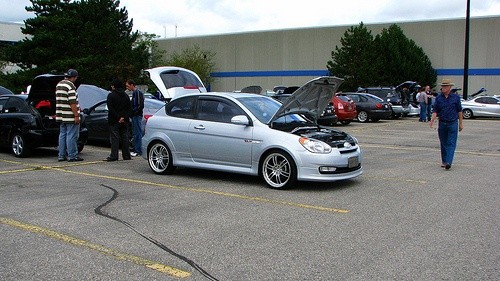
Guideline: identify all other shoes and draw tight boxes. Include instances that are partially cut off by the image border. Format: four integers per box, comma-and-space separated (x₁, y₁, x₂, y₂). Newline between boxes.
441, 163, 445, 167
102, 157, 114, 161
132, 153, 139, 157
69, 156, 83, 161
130, 152, 134, 155
445, 163, 451, 169
58, 157, 69, 162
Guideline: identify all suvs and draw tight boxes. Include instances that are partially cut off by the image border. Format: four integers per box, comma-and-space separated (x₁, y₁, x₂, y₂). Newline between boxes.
355, 80, 418, 120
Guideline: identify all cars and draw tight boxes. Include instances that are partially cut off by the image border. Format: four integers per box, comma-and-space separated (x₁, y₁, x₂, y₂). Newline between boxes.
140, 76, 363, 190
70, 84, 134, 148
123, 66, 208, 137
330, 92, 356, 126
460, 96, 500, 119
0, 86, 16, 112
234, 83, 338, 127
0, 74, 88, 157
342, 92, 393, 123
407, 93, 420, 117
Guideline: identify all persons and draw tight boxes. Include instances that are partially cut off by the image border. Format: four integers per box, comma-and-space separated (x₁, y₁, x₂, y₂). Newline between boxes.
126, 79, 144, 156
103, 79, 132, 161
55, 68, 83, 162
429, 79, 464, 170
397, 84, 438, 123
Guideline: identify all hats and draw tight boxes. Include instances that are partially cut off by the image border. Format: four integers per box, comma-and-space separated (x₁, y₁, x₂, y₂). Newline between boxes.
438, 79, 455, 86
64, 68, 78, 76
